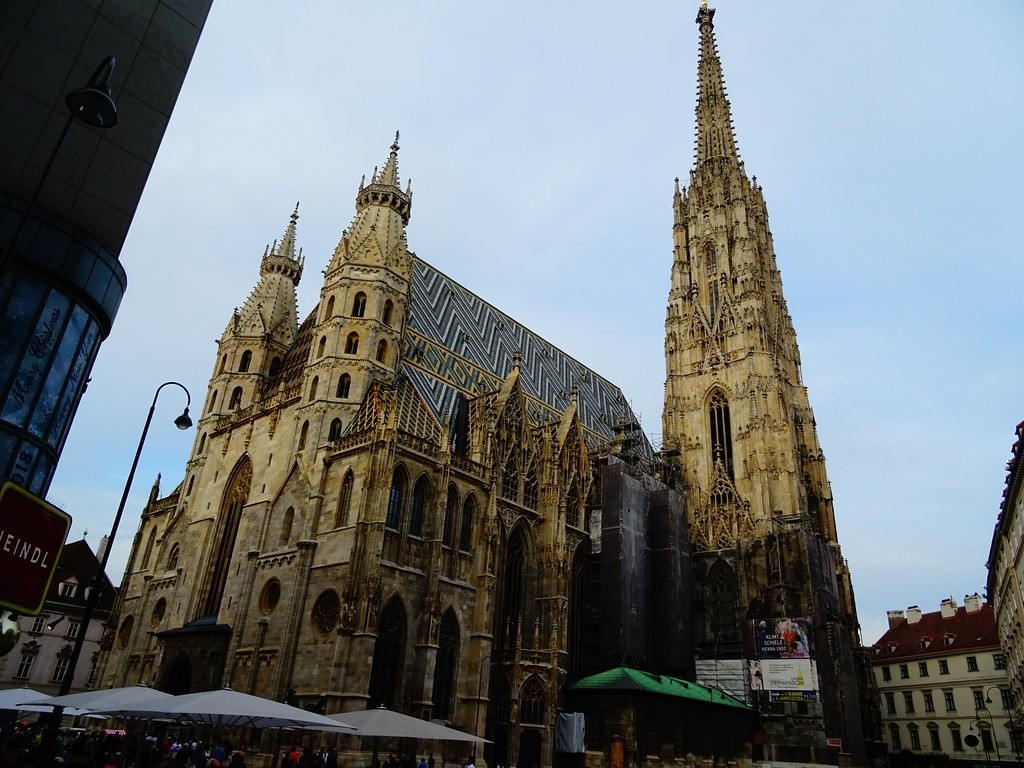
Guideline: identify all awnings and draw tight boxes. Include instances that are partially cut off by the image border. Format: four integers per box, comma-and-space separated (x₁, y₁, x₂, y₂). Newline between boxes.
570, 667, 756, 711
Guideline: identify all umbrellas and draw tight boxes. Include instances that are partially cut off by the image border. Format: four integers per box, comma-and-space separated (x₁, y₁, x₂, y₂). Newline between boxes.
288, 704, 494, 768
80, 683, 356, 748
15, 681, 176, 713
0, 684, 113, 728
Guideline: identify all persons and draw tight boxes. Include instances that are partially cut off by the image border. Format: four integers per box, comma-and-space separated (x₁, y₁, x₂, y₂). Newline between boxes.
0, 729, 475, 768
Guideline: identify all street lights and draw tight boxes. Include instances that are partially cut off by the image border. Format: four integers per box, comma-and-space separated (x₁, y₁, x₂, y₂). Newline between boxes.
473, 656, 514, 766
969, 720, 988, 761
986, 686, 1021, 762
975, 708, 1000, 761
39, 382, 193, 768
